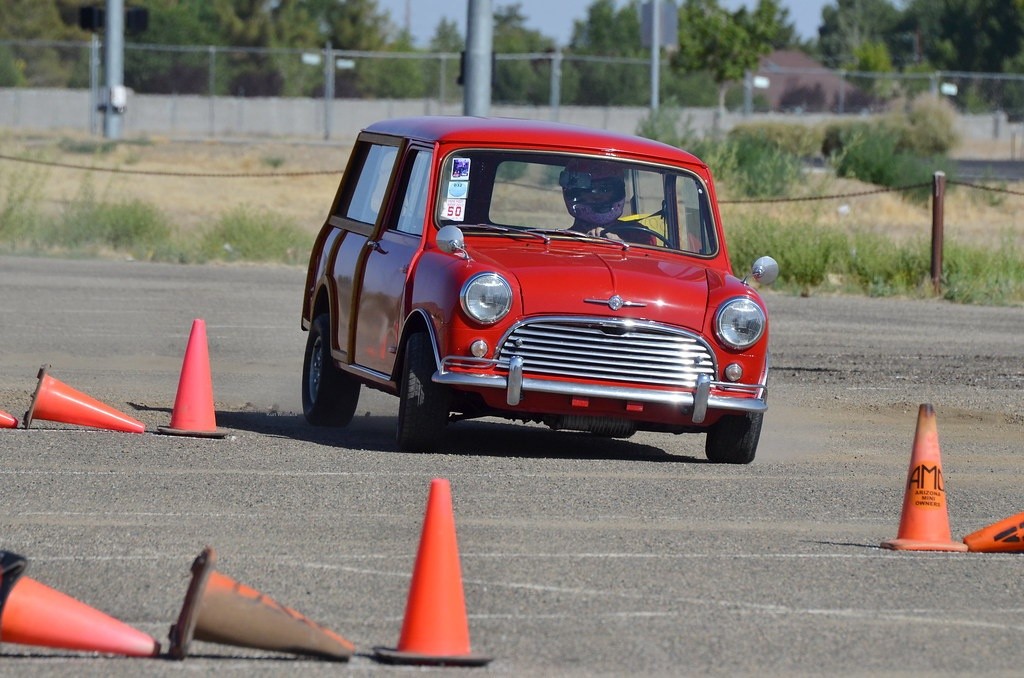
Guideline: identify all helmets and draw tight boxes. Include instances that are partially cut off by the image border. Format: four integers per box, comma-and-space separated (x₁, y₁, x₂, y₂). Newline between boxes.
559, 158, 627, 225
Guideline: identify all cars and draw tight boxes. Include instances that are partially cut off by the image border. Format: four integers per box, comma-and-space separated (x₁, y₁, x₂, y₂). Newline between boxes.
301, 116, 778, 464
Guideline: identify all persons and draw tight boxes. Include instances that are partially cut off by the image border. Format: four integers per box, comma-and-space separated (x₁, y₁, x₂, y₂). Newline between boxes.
556, 159, 655, 247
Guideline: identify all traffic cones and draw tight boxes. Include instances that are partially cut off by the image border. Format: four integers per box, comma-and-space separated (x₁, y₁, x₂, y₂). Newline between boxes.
880, 404, 969, 552
22, 363, 146, 434
964, 512, 1024, 552
0, 577, 161, 658
166, 547, 356, 662
158, 319, 229, 438
374, 478, 496, 668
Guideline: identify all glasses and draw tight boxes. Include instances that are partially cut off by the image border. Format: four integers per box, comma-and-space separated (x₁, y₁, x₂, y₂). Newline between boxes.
574, 183, 618, 194
